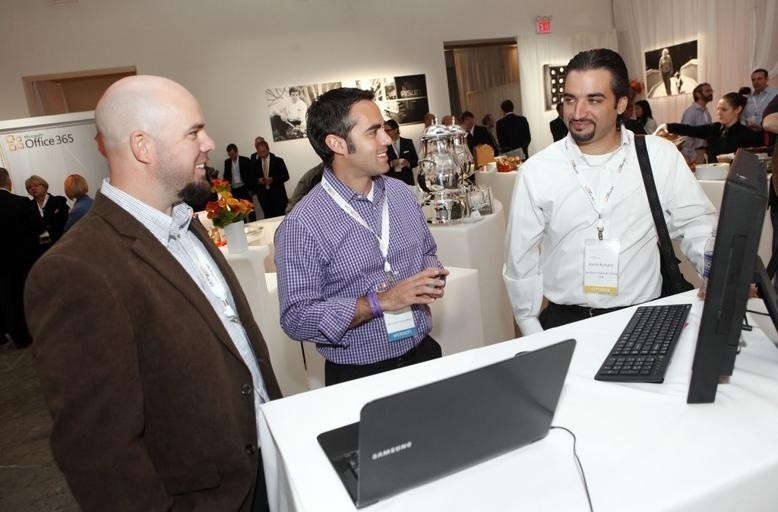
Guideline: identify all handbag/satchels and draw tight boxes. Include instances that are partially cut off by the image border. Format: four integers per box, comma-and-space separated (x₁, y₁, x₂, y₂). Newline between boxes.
659, 263, 695, 297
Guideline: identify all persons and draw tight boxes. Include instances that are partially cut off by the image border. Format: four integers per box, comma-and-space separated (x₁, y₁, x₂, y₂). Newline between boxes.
0, 168, 93, 350
501, 49, 757, 335
760, 92, 777, 290
272, 89, 450, 387
625, 69, 778, 166
659, 48, 673, 95
271, 86, 309, 139
184, 138, 321, 228
382, 97, 569, 186
24, 74, 285, 512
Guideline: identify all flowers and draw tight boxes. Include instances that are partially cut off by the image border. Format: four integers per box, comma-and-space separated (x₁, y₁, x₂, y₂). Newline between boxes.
203, 173, 256, 230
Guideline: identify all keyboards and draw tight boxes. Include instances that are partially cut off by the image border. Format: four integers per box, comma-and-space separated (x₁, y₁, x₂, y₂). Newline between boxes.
595, 303, 692, 383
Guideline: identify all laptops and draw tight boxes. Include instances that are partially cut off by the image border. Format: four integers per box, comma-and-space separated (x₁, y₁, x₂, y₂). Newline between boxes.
317, 337, 577, 510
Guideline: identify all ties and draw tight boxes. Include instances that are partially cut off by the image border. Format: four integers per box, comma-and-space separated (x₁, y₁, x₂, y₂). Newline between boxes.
263, 158, 268, 179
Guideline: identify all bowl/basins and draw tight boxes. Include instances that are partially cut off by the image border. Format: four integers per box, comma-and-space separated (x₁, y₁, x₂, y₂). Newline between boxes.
695, 163, 729, 180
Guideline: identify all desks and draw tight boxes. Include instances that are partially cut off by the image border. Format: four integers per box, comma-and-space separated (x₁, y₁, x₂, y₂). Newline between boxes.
196, 146, 776, 411
252, 280, 777, 512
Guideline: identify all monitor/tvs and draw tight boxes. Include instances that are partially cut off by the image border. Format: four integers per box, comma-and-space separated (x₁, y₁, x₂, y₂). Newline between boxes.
687, 148, 778, 404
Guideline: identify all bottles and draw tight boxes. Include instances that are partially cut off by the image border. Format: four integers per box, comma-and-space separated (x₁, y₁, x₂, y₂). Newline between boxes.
703, 226, 719, 299
212, 228, 221, 245
